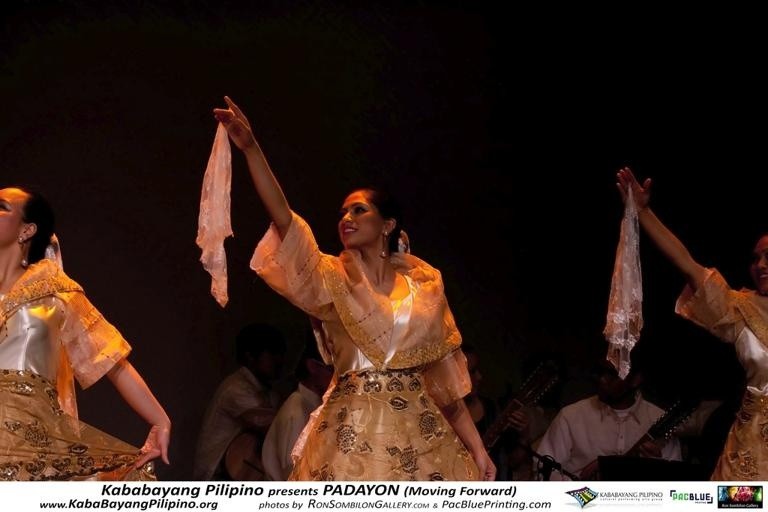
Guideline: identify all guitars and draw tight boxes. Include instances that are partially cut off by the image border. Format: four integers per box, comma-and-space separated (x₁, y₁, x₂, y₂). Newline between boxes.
224, 431, 265, 483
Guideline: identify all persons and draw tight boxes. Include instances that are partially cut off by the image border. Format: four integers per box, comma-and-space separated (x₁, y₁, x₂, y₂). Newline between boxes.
190, 320, 288, 481
259, 339, 333, 481
0, 182, 172, 481
458, 344, 541, 482
210, 94, 502, 481
615, 164, 767, 482
530, 350, 683, 481
